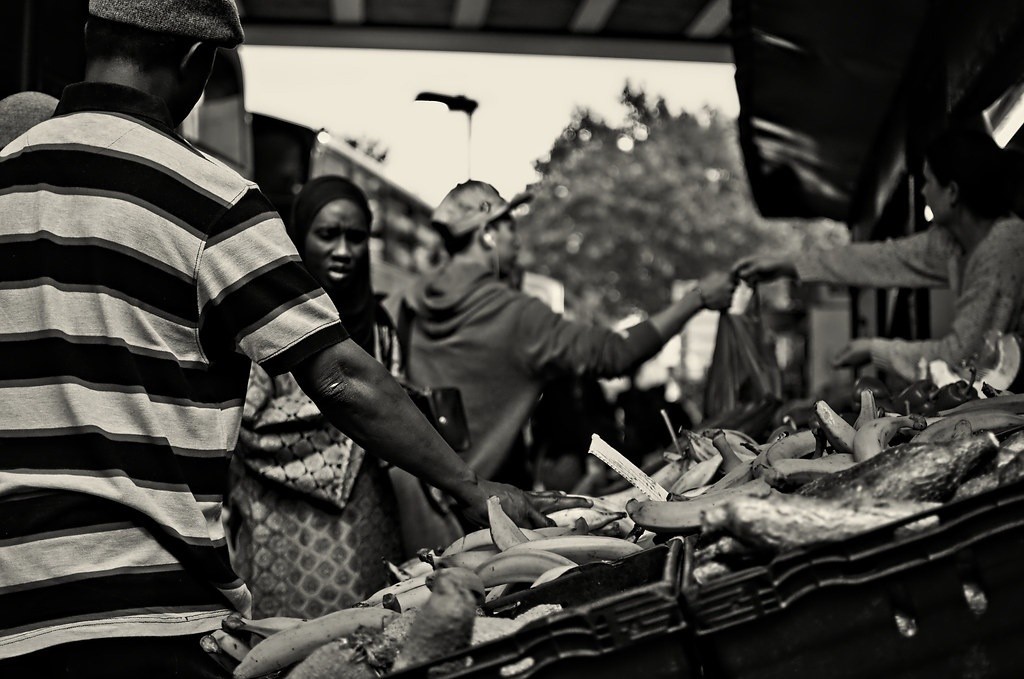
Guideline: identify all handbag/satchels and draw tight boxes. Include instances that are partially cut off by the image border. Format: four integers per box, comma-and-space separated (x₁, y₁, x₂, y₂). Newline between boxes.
704, 278, 784, 423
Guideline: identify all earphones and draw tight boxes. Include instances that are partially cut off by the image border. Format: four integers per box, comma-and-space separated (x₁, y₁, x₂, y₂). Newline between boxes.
482, 233, 496, 247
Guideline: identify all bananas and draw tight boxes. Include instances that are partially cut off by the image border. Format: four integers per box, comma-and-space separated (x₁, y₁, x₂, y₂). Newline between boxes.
200, 391, 1024, 679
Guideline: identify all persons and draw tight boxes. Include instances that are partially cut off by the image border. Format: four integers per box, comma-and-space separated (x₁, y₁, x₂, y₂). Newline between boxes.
382, 177, 739, 536
0, 0, 594, 679
730, 124, 1024, 394
233, 175, 412, 618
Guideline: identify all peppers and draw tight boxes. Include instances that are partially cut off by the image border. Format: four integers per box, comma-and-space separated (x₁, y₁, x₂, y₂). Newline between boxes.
576, 362, 979, 497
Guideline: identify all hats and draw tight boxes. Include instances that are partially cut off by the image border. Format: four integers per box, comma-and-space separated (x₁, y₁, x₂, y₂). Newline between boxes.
433, 179, 534, 236
88, 0, 244, 50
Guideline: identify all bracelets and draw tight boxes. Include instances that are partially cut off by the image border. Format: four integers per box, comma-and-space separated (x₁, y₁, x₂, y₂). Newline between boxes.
692, 286, 707, 307
